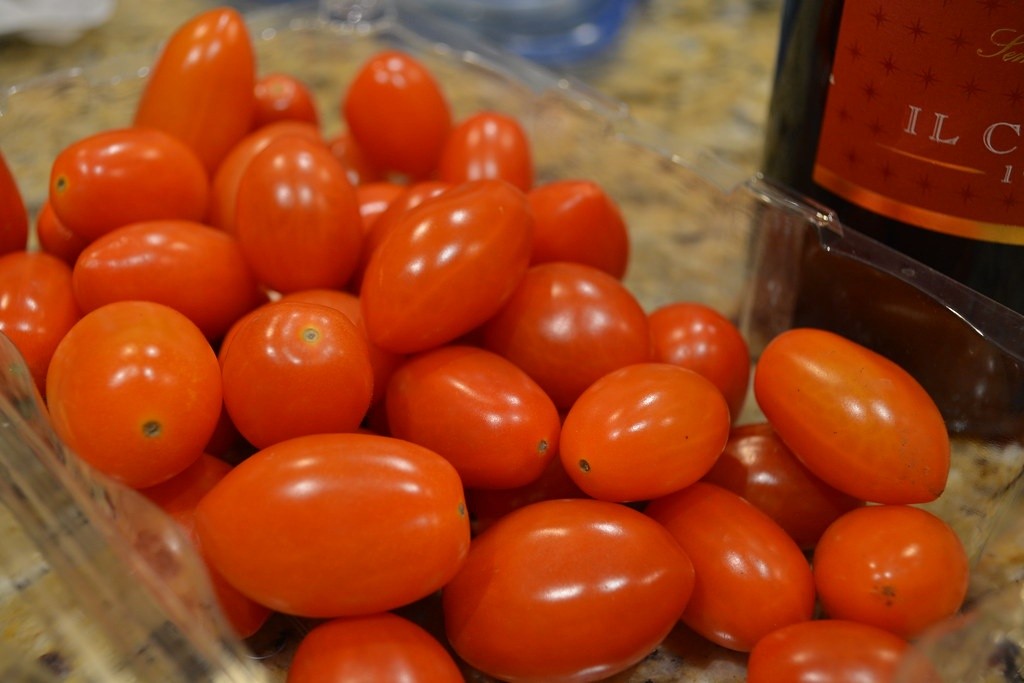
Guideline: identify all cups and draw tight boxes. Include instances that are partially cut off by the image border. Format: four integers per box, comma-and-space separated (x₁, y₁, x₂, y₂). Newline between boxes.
738, 0, 1024, 443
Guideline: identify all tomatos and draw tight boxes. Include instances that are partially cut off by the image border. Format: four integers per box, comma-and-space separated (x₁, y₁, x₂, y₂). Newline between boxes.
1, 9, 970, 683
